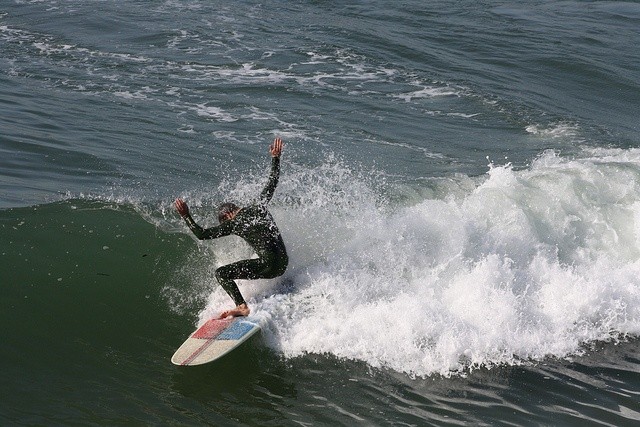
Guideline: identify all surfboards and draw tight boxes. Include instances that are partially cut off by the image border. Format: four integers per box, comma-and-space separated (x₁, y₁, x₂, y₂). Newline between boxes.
172, 318, 261, 366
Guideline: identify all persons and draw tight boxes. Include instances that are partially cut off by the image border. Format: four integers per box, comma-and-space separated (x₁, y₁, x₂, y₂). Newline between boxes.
173, 138, 288, 321
